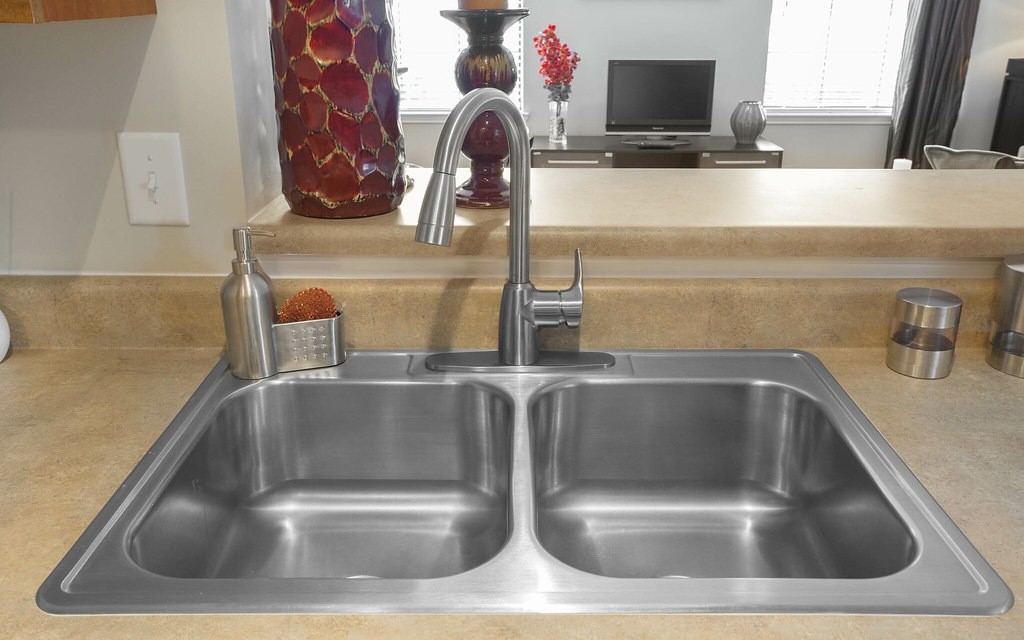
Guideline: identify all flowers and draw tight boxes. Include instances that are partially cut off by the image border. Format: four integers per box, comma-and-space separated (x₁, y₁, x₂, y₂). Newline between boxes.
534, 24, 580, 101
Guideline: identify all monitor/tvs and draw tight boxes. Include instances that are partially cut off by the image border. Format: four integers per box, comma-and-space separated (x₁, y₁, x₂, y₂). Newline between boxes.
605, 60, 716, 144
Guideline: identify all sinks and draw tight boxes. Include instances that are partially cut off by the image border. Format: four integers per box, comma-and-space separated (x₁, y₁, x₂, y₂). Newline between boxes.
526, 348, 1014, 615
36, 352, 514, 615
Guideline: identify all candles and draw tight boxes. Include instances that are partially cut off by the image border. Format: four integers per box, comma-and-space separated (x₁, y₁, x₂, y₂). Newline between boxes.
459, 0, 509, 10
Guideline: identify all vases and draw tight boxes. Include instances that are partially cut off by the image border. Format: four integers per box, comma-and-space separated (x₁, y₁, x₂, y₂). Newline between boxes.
729, 101, 767, 145
549, 101, 568, 142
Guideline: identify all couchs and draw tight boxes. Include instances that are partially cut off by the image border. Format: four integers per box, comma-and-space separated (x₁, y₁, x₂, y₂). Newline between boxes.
924, 144, 1017, 169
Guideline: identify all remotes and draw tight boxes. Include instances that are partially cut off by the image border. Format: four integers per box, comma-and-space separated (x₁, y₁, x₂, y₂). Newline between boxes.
637, 141, 675, 149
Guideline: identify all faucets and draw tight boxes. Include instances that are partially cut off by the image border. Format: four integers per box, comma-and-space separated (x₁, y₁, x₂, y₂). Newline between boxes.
413, 87, 618, 374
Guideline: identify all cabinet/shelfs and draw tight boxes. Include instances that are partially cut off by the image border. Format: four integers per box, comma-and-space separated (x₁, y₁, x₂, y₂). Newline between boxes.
989, 59, 1024, 155
530, 134, 783, 168
